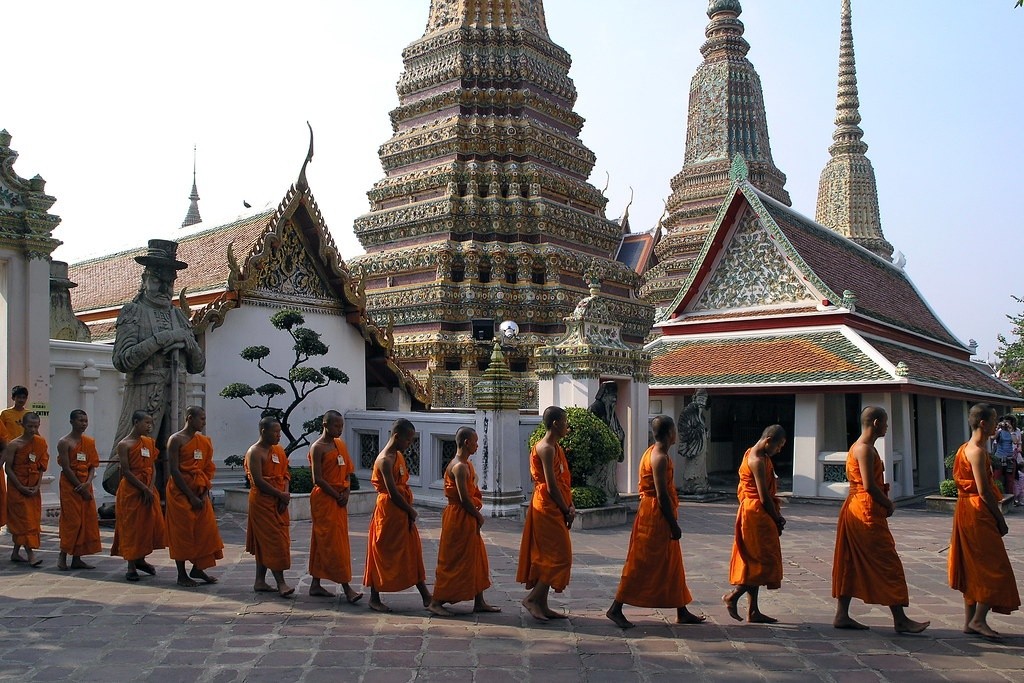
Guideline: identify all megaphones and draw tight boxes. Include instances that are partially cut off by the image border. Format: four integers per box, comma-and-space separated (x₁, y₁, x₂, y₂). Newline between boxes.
499, 321, 519, 340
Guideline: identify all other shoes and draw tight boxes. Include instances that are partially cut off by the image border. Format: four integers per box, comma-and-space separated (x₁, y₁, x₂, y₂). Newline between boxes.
1019, 501, 1024, 505
1014, 501, 1019, 506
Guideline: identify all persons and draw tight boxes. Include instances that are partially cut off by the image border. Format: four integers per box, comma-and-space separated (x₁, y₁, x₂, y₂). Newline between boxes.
606, 414, 707, 628
991, 413, 1024, 507
948, 403, 1021, 638
98, 238, 206, 518
362, 418, 434, 612
308, 409, 364, 603
678, 388, 712, 496
164, 405, 224, 587
428, 425, 501, 617
722, 424, 787, 622
0, 385, 49, 566
110, 410, 168, 582
587, 381, 625, 506
57, 409, 103, 571
832, 406, 930, 633
515, 406, 576, 621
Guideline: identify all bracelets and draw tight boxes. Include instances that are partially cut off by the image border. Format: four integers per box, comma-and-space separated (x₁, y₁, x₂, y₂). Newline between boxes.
1010, 432, 1017, 435
